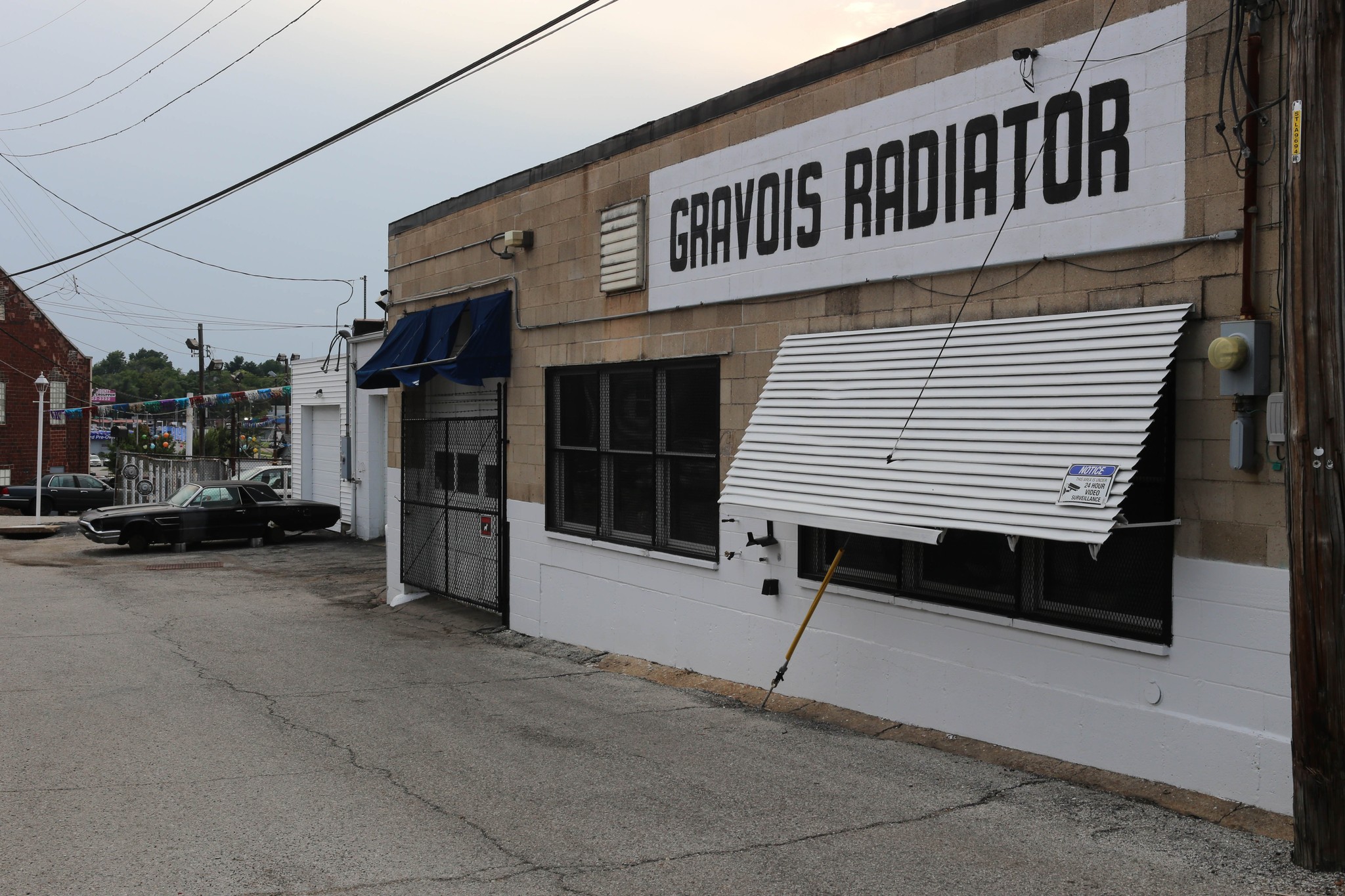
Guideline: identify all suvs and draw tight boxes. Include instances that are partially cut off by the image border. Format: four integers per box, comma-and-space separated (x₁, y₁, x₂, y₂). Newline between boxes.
188, 463, 291, 502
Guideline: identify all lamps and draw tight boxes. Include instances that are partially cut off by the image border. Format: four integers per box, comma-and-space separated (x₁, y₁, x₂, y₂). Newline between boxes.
504, 228, 534, 249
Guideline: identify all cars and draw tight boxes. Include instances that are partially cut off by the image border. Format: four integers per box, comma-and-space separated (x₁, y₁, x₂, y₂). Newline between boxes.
90, 454, 102, 467
90, 472, 115, 488
103, 458, 112, 466
77, 480, 343, 554
0, 473, 147, 516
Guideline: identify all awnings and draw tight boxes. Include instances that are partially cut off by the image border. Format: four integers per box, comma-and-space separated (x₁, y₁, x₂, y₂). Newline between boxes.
356, 286, 514, 392
716, 302, 1195, 547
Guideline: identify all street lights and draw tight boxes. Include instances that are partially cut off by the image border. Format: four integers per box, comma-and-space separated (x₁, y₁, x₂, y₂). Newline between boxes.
157, 420, 163, 436
275, 353, 301, 434
230, 371, 243, 456
34, 371, 50, 516
130, 411, 148, 448
185, 322, 224, 458
268, 370, 278, 448
